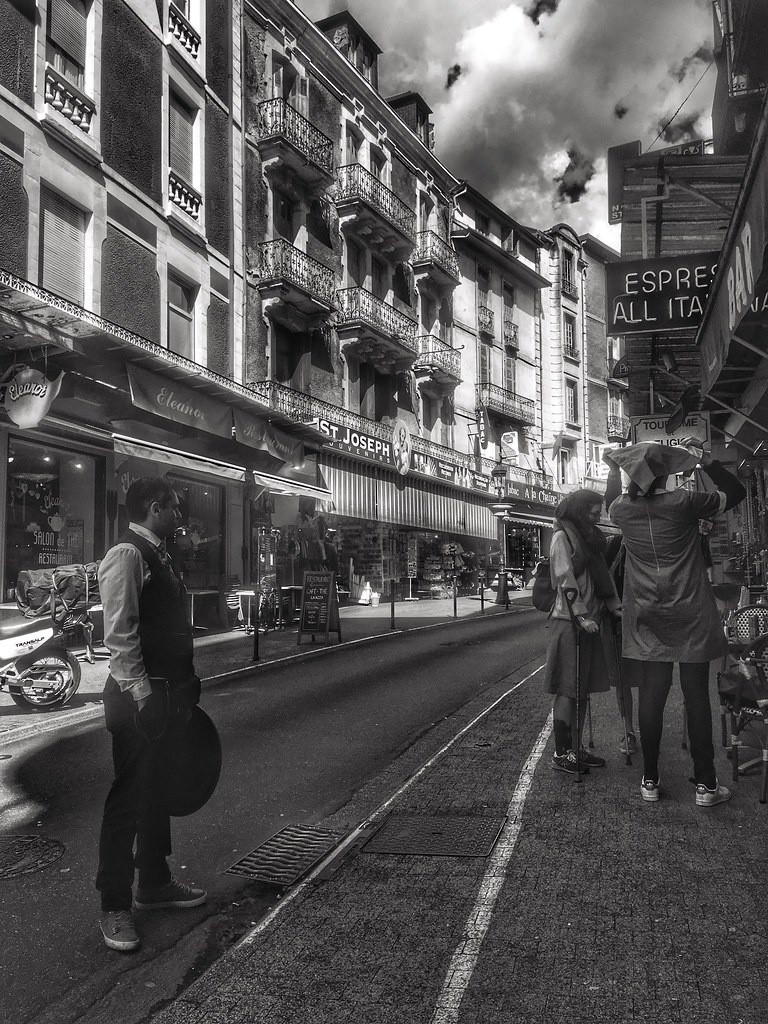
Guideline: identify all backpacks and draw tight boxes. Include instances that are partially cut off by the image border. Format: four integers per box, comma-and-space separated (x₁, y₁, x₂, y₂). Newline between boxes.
532, 529, 574, 612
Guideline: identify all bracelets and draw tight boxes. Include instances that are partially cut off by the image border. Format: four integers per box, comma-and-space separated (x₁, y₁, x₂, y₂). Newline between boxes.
578, 615, 588, 623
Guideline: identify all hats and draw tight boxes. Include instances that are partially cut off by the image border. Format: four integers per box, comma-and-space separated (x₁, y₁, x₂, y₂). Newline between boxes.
151, 704, 220, 815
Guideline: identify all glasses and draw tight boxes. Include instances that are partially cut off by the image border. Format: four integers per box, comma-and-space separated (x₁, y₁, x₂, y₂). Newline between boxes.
586, 512, 599, 517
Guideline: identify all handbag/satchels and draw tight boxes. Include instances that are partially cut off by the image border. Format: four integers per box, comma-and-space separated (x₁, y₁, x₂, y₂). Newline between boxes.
716, 669, 762, 706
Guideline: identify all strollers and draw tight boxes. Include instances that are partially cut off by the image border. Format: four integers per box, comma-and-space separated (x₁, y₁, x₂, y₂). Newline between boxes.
717, 606, 768, 802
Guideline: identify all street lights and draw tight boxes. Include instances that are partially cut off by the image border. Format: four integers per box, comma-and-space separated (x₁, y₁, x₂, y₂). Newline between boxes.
491, 462, 514, 605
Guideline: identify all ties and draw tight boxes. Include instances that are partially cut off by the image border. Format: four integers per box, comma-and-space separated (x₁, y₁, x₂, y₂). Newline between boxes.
154, 544, 180, 609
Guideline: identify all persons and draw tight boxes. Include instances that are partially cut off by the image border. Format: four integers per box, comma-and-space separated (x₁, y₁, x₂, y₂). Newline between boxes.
602, 532, 641, 755
543, 489, 607, 775
602, 435, 746, 806
94, 475, 210, 953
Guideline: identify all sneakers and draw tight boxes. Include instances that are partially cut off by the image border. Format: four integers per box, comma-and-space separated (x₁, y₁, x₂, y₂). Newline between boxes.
99, 910, 140, 951
620, 731, 637, 754
694, 778, 731, 807
135, 880, 208, 910
640, 775, 662, 802
552, 748, 605, 774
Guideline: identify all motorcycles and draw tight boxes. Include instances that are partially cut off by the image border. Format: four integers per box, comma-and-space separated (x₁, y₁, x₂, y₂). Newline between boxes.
0, 607, 95, 714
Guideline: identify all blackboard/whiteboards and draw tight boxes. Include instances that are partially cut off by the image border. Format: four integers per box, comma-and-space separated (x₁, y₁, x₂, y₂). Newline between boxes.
298, 571, 341, 634
